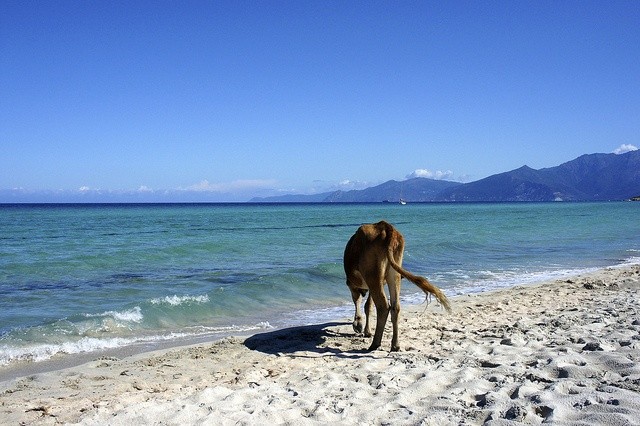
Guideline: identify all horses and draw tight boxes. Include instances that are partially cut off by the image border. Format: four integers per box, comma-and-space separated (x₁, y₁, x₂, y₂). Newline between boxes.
343, 219, 452, 350
